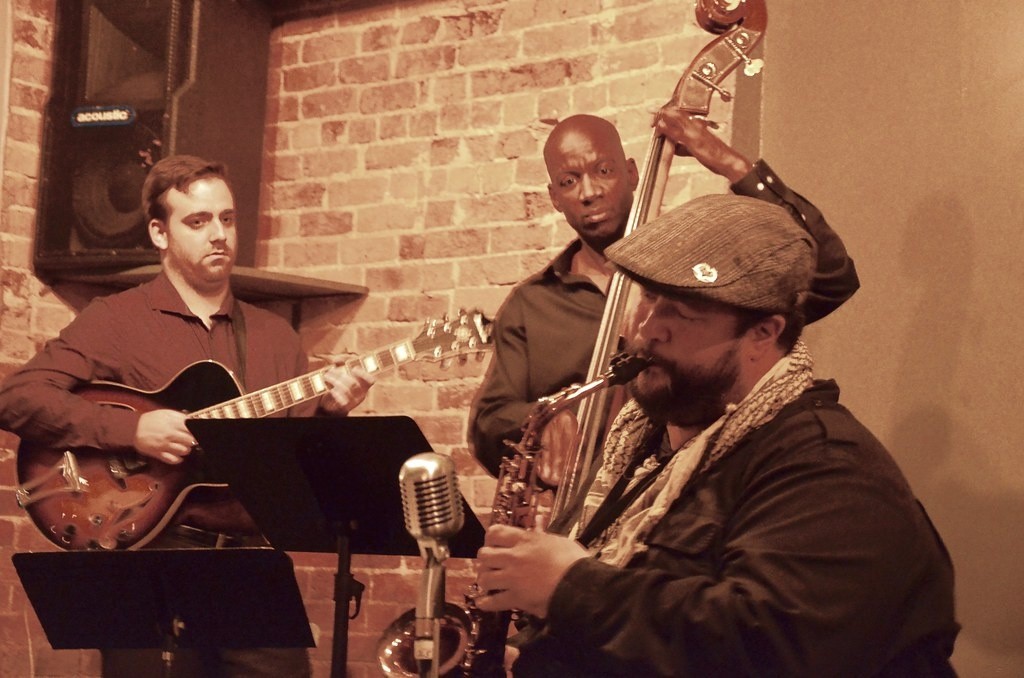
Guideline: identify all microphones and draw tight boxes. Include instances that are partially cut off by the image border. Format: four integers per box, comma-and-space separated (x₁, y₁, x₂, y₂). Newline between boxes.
397, 453, 467, 565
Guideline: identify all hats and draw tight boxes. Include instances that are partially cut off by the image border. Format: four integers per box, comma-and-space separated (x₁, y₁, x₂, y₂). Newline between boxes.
600, 194, 818, 312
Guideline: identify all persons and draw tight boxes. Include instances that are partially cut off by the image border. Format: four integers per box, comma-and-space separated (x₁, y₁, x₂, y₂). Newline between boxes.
2, 152, 376, 678
467, 194, 961, 678
465, 114, 859, 488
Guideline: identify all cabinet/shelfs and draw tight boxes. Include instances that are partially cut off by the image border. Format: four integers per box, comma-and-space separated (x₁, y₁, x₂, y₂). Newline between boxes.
70, 262, 368, 334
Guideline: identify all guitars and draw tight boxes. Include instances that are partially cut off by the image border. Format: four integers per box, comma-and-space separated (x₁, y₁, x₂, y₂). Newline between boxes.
13, 306, 495, 552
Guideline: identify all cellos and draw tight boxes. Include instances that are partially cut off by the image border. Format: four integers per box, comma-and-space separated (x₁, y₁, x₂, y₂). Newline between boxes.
515, 0, 769, 539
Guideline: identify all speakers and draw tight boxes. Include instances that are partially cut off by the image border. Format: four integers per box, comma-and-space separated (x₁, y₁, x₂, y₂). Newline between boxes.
31, 1, 273, 285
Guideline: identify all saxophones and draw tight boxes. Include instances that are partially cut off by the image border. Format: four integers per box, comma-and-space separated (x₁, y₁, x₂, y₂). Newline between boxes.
379, 345, 657, 677
